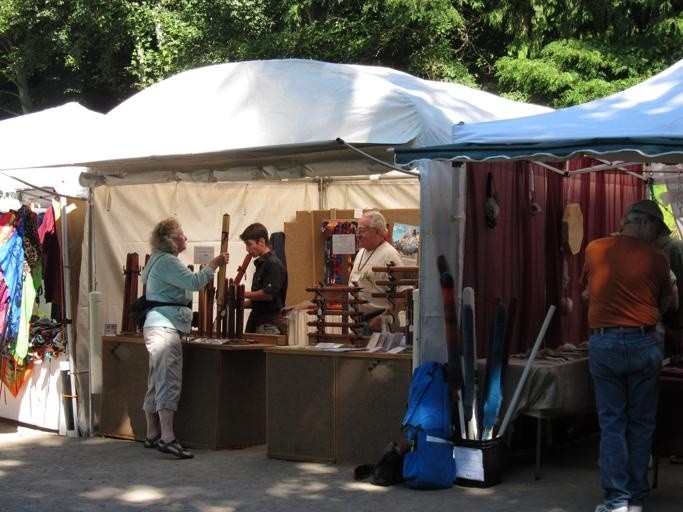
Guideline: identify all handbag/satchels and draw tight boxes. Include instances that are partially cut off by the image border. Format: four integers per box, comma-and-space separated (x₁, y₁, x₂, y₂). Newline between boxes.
354, 444, 412, 485
131, 294, 148, 329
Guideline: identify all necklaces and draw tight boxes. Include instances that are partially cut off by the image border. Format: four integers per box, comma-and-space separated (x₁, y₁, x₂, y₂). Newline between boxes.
358, 241, 384, 272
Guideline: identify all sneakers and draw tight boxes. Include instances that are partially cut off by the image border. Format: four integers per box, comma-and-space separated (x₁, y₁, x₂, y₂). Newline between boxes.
630, 499, 648, 512
594, 499, 628, 512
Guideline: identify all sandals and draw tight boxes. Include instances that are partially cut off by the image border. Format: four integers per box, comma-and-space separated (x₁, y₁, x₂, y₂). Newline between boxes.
155, 437, 195, 458
144, 432, 161, 448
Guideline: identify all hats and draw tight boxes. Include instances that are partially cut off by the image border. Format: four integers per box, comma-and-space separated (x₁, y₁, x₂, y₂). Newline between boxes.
631, 199, 671, 237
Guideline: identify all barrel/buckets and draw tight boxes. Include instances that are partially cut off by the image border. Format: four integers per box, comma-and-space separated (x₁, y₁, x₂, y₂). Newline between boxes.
451, 435, 498, 488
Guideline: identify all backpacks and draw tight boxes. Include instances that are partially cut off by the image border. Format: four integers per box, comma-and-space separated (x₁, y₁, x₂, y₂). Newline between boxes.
399, 360, 457, 490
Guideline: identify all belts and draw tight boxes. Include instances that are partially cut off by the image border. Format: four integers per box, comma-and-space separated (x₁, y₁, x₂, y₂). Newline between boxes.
592, 325, 655, 334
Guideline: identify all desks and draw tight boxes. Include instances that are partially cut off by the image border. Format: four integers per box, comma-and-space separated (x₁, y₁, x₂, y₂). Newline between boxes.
652, 376, 683, 489
262, 346, 413, 464
476, 344, 588, 482
102, 334, 274, 450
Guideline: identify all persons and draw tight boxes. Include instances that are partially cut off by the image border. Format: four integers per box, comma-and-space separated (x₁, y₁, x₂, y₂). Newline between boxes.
348, 210, 402, 335
240, 222, 289, 336
576, 196, 672, 512
652, 221, 682, 467
140, 217, 229, 458
